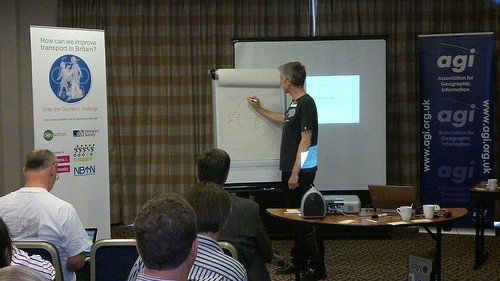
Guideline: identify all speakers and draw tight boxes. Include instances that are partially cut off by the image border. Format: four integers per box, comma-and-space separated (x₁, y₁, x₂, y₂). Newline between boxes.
301, 187, 326, 218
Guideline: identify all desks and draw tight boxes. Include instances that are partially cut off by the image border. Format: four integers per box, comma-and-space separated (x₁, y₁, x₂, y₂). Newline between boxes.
468, 180, 500, 270
266, 208, 468, 281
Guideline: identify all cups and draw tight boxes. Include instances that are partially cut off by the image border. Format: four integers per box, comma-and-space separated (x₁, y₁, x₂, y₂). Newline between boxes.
423, 205, 435, 218
488, 179, 497, 190
396, 206, 412, 221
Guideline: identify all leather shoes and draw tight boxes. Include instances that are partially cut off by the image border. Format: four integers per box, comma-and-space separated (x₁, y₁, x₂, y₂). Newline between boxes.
295, 266, 326, 281
276, 262, 309, 275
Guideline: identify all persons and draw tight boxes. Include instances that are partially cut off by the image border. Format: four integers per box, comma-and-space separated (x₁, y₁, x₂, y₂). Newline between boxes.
0, 149, 94, 281
197, 148, 274, 281
0, 217, 12, 268
128, 181, 248, 281
134, 192, 198, 281
247, 61, 327, 281
10, 245, 56, 280
0, 265, 49, 281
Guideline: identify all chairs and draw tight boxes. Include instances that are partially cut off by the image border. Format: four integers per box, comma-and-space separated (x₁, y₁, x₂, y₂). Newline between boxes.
89, 240, 237, 280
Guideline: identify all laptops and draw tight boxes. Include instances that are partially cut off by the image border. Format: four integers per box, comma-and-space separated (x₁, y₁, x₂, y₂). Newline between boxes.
368, 185, 423, 213
83, 228, 98, 262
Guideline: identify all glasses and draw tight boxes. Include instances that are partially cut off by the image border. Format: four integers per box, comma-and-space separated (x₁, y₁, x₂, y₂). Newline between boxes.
55, 167, 59, 181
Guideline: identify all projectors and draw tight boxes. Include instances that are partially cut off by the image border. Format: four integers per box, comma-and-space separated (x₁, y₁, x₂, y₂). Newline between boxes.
323, 195, 361, 214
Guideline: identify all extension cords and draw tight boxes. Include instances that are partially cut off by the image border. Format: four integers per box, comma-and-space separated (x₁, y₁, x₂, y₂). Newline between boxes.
361, 208, 374, 213
273, 253, 284, 260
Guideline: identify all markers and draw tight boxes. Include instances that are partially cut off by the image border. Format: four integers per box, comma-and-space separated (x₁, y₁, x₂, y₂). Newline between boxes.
247, 98, 257, 103
371, 213, 387, 218
